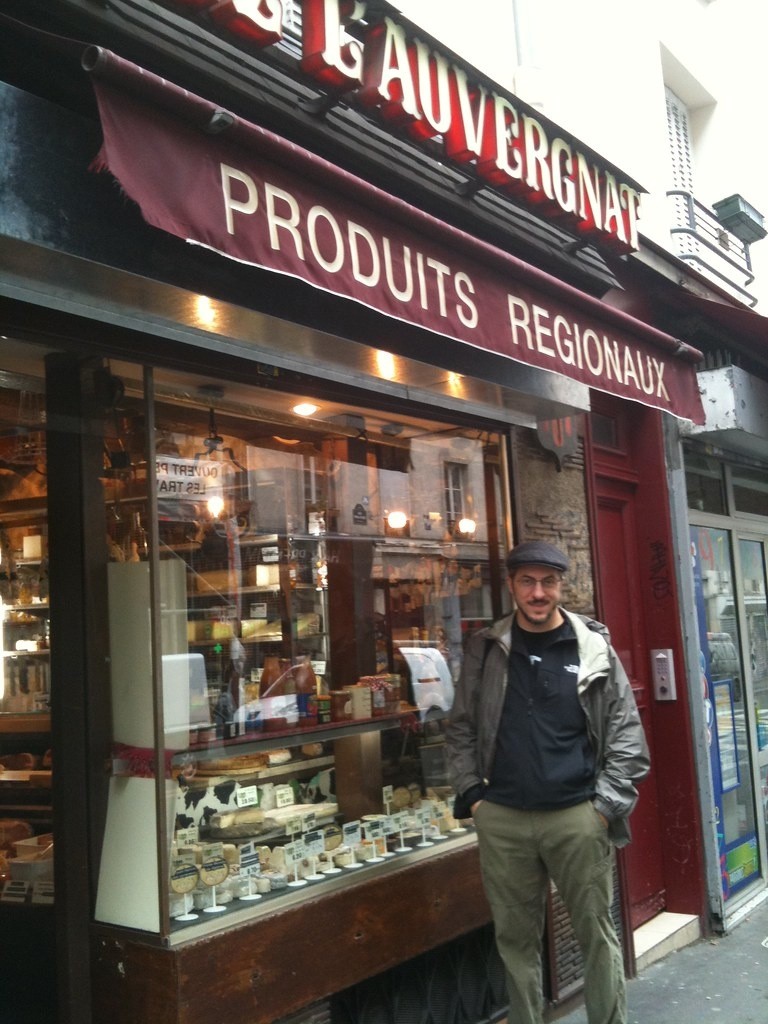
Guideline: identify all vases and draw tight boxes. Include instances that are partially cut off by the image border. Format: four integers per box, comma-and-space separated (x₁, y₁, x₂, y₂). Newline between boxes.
712, 194, 768, 245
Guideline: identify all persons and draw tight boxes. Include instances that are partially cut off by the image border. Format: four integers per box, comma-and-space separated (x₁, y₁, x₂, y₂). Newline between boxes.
445, 541, 651, 1024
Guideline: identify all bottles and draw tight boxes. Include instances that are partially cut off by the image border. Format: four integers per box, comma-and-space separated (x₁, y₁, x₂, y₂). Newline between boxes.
296, 655, 318, 726
281, 659, 299, 728
244, 679, 263, 734
421, 630, 442, 708
407, 626, 429, 706
224, 654, 244, 712
259, 652, 286, 730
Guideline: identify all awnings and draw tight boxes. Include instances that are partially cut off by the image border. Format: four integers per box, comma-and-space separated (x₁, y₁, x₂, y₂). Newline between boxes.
0, 11, 708, 426
670, 289, 768, 363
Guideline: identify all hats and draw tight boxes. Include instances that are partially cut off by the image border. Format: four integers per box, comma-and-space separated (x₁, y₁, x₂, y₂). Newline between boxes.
505, 540, 568, 573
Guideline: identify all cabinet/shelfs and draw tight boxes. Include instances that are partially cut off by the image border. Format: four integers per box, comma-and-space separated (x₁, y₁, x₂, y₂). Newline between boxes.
0, 467, 507, 918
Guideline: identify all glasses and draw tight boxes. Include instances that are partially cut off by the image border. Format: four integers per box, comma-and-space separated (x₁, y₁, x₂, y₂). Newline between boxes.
512, 575, 562, 590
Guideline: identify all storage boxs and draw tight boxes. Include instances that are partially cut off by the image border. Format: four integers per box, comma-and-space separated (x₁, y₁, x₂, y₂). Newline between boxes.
21, 536, 47, 559
10, 829, 54, 857
247, 561, 298, 587
8, 851, 54, 882
196, 569, 243, 591
187, 619, 218, 640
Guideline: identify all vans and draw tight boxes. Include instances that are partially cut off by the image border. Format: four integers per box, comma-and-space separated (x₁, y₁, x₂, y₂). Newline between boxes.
708, 634, 742, 702
231, 647, 455, 736
